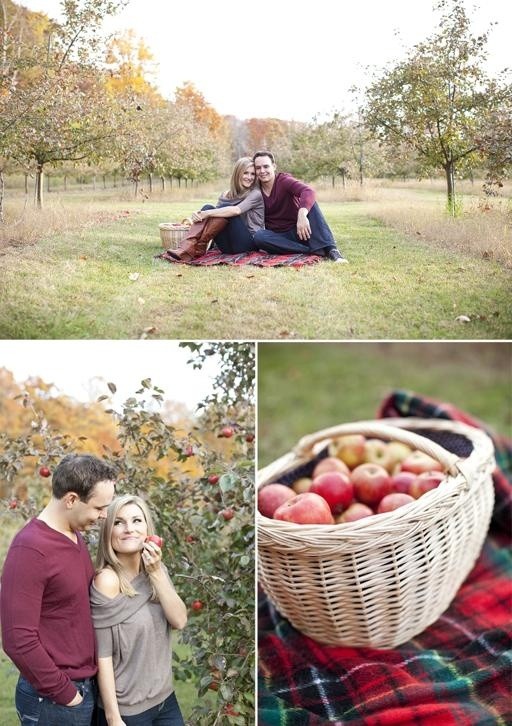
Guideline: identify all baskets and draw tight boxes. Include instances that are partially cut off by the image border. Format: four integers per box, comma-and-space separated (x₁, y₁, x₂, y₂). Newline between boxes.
159, 218, 192, 250
257, 418, 496, 651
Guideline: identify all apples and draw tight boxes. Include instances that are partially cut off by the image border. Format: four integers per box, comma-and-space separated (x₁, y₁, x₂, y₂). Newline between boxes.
39, 466, 50, 478
185, 426, 255, 716
9, 500, 18, 509
146, 535, 161, 549
258, 436, 448, 523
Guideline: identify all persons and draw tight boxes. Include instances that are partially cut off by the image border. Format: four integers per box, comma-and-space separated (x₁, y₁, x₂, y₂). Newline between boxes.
89, 494, 188, 726
167, 156, 265, 261
0, 452, 115, 725
253, 151, 349, 264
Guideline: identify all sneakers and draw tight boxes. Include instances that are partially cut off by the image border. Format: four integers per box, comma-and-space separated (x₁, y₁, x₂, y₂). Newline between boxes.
330, 249, 347, 263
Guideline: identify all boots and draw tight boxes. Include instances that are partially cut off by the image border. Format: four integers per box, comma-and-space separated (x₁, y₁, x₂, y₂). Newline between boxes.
167, 214, 228, 260
